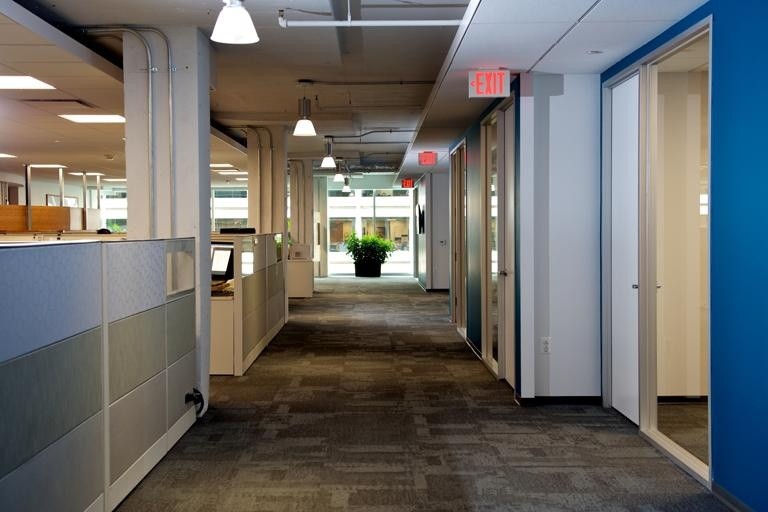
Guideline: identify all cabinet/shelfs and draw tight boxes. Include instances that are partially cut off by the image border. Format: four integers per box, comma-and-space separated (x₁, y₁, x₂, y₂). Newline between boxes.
210, 229, 289, 378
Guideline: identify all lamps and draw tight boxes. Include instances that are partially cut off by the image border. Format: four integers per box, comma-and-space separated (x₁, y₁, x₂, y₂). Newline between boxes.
333, 165, 345, 182
291, 86, 318, 137
321, 133, 337, 168
208, 1, 261, 46
342, 177, 351, 193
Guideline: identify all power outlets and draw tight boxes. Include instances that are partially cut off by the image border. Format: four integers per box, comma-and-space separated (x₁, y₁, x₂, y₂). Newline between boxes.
540, 336, 551, 353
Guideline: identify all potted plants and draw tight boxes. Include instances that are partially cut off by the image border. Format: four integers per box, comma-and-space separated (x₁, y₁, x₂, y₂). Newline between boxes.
342, 229, 398, 277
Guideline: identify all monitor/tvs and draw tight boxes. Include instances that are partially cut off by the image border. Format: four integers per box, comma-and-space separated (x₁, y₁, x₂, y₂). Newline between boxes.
210, 242, 234, 279
